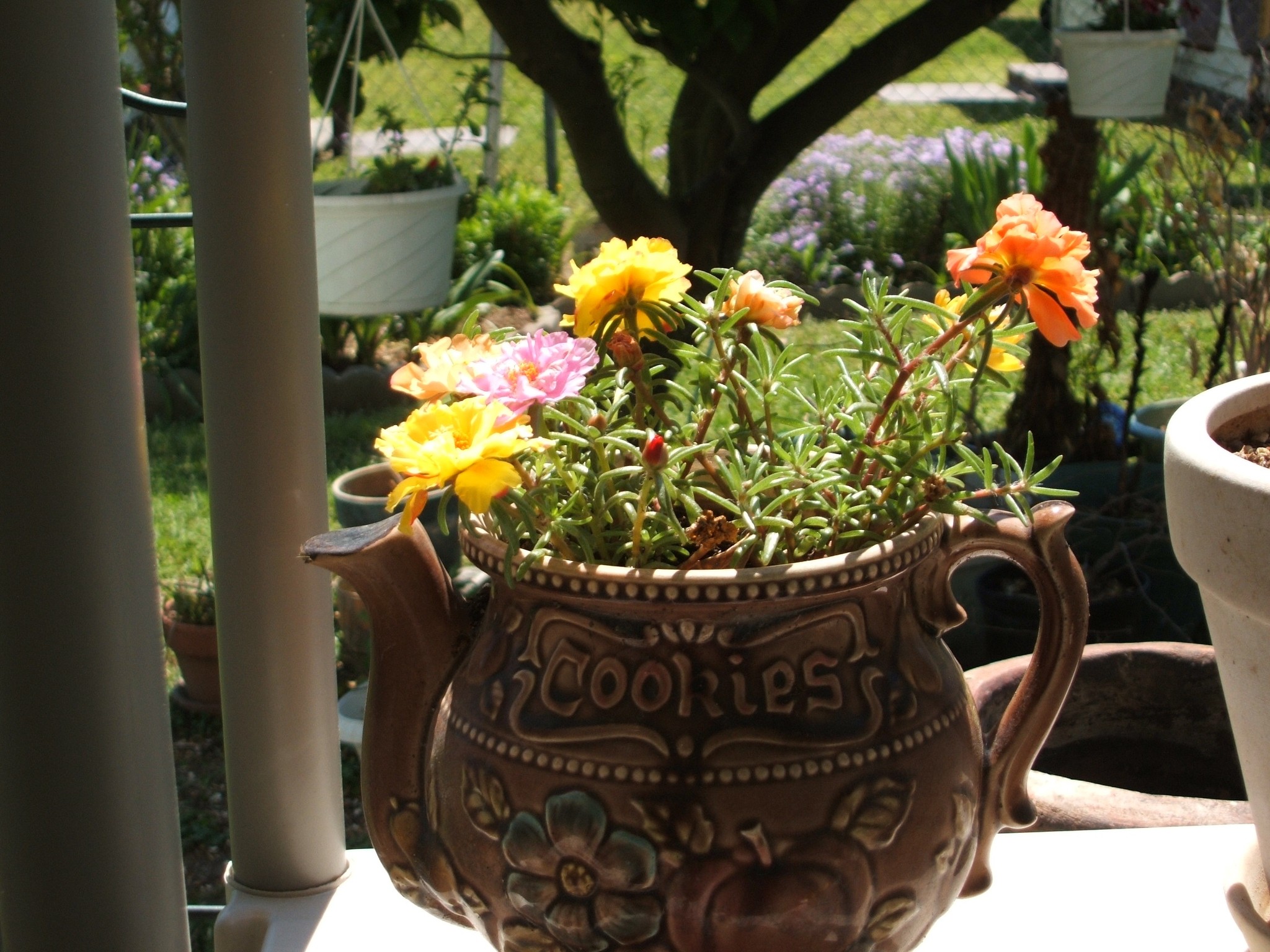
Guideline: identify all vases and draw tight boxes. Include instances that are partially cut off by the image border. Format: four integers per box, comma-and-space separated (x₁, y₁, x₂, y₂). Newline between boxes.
1161, 373, 1270, 928
1062, 36, 1174, 119
296, 501, 1087, 952
313, 198, 455, 315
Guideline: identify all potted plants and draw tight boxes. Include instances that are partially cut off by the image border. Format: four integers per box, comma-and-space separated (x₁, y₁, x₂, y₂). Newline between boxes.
1129, 39, 1270, 479
156, 546, 219, 717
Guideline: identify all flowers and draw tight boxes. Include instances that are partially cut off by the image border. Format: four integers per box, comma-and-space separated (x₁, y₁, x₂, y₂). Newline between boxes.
1053, 1, 1209, 32
311, 67, 502, 196
373, 191, 1103, 593
